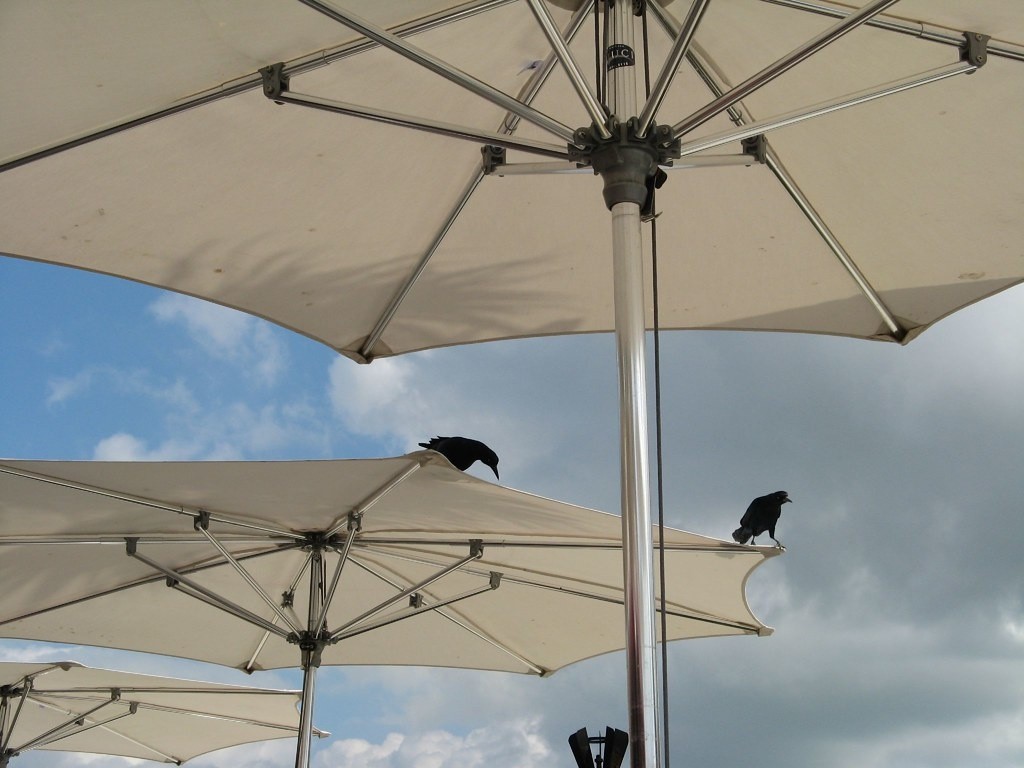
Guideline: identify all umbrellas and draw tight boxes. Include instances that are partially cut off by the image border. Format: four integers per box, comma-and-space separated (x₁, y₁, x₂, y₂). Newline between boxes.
0, 449, 786, 768
0, 0, 1024, 768
0, 660, 332, 768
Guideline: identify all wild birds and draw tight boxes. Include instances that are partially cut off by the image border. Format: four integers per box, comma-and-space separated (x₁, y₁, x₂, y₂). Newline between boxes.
417, 435, 500, 483
730, 490, 794, 551
